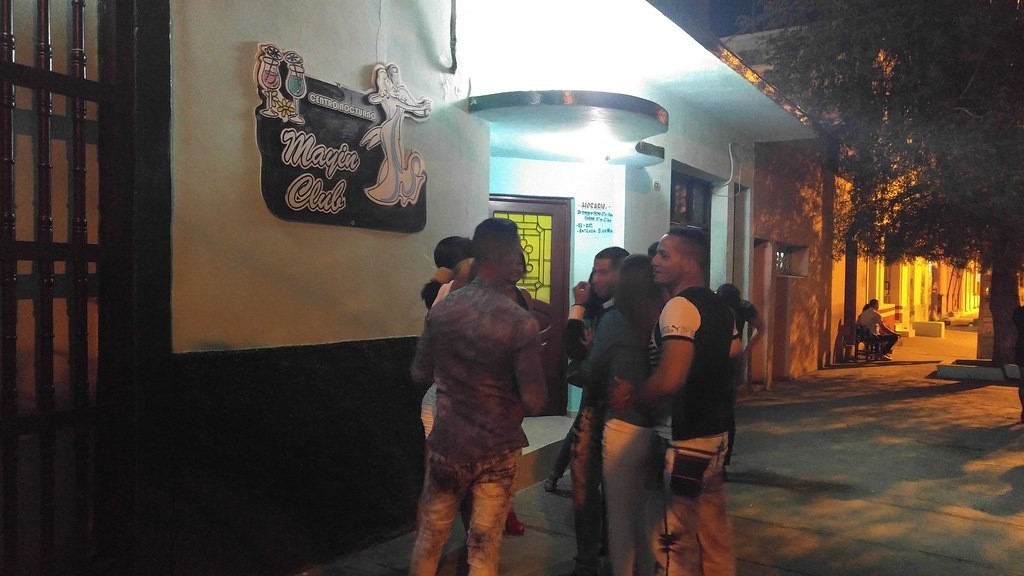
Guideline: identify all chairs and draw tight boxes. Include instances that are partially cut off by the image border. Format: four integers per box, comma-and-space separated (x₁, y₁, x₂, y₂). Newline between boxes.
854, 325, 881, 362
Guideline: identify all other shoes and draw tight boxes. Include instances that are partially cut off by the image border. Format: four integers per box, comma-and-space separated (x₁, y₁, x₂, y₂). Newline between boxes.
572, 543, 607, 561
879, 354, 892, 361
544, 479, 556, 493
504, 512, 525, 534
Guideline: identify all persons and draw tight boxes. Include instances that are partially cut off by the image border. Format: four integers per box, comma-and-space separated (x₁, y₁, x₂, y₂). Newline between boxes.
856, 299, 901, 361
544, 226, 765, 576
1014, 305, 1024, 421
406, 216, 552, 576
